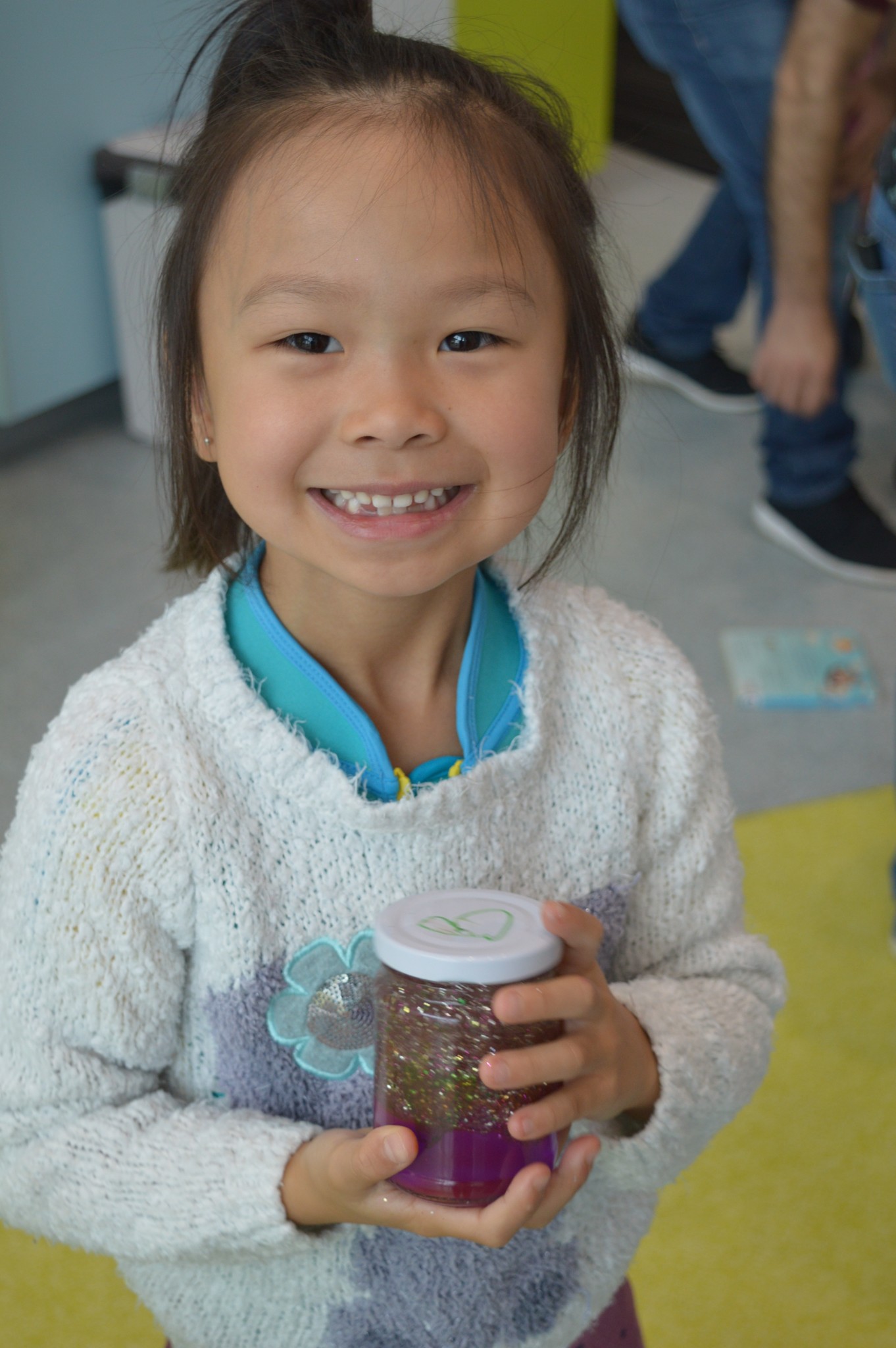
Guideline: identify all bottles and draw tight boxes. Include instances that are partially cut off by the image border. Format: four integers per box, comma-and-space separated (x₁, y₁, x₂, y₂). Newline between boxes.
374, 888, 566, 1209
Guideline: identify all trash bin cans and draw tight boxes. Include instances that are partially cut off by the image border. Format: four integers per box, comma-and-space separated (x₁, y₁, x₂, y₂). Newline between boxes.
94, 111, 210, 443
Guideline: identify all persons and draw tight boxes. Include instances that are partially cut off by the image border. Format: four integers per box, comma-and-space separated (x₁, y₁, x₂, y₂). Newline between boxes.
613, 0, 895, 585
0, 1, 785, 1348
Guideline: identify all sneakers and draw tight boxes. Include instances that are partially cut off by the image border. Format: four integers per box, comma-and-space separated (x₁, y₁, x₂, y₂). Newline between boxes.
749, 479, 896, 583
624, 322, 765, 415
